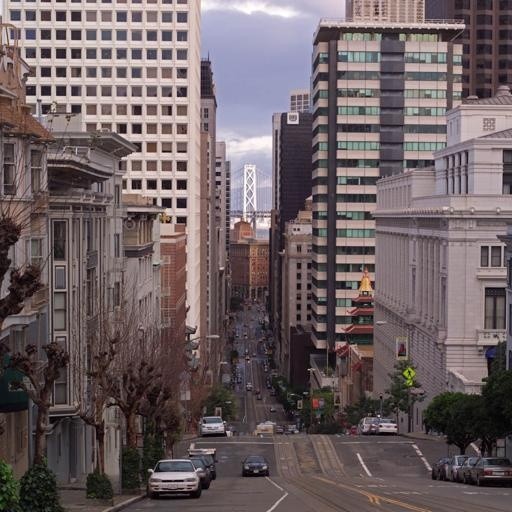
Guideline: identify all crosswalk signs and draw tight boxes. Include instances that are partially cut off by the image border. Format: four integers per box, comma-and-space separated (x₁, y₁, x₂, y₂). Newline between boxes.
403, 367, 416, 379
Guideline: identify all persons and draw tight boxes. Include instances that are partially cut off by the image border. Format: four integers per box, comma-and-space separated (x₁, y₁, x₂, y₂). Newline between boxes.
422, 417, 429, 434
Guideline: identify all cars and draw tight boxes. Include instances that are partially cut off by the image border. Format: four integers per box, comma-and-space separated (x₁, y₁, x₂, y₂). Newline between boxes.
349, 416, 398, 435
198, 298, 306, 435
241, 455, 270, 477
148, 448, 220, 500
432, 455, 512, 486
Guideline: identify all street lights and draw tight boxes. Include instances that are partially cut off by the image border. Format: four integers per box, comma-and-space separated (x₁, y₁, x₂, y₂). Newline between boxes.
182, 335, 220, 344
